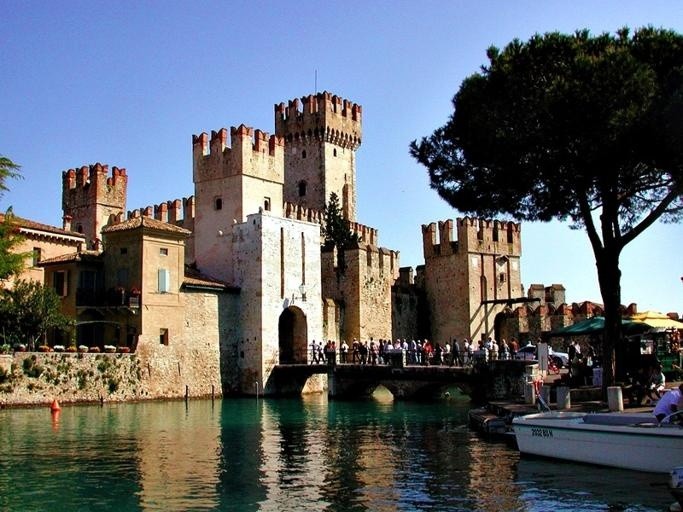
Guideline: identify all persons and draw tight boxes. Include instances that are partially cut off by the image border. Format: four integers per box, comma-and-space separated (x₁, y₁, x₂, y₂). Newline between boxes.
567, 339, 596, 363
646, 364, 666, 402
89, 236, 102, 250
653, 384, 683, 422
309, 337, 541, 365
548, 343, 553, 361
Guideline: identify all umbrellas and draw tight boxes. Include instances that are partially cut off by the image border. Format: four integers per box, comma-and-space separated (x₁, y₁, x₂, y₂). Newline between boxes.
551, 316, 656, 337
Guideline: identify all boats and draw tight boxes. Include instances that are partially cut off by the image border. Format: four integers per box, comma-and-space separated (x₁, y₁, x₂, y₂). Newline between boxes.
513, 411, 683, 472
